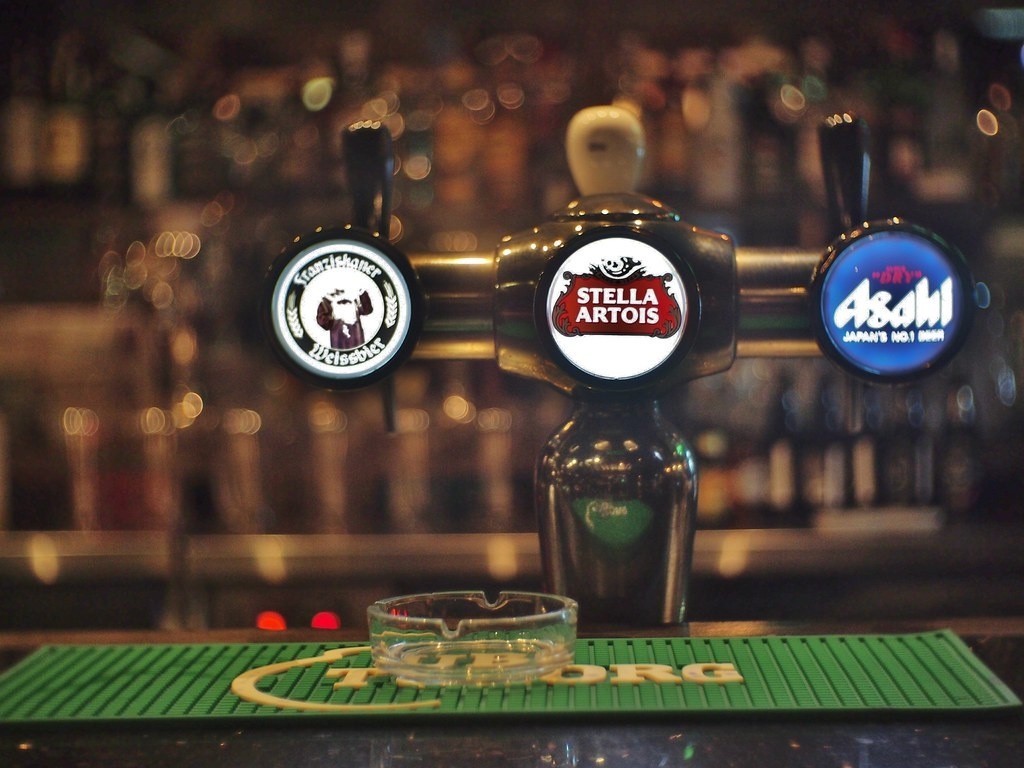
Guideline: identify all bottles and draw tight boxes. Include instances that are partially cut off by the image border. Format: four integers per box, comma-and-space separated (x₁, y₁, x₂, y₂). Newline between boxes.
0, 18, 1023, 241
684, 357, 988, 525
